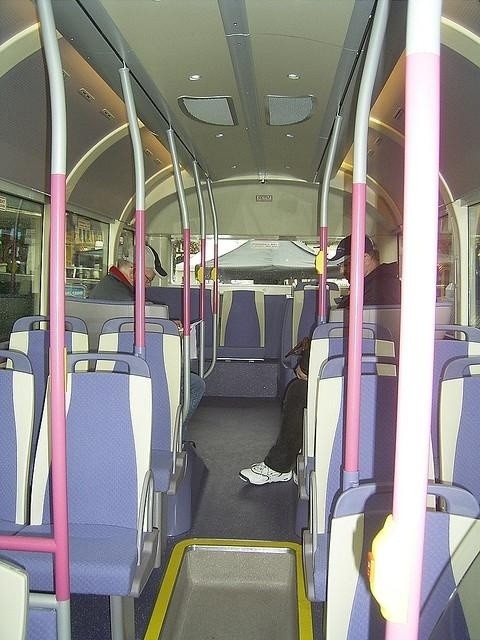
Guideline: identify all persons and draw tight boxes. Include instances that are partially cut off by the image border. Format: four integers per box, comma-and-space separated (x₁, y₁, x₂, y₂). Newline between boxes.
239, 232, 403, 486
88, 244, 205, 429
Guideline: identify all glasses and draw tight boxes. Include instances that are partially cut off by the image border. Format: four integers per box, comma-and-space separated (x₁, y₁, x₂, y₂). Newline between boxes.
131, 267, 153, 284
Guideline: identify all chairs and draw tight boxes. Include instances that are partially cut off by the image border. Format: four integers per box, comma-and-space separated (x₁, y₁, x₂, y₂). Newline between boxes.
0, 278, 480, 640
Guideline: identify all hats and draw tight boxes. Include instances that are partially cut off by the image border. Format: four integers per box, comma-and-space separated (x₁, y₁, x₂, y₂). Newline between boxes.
327, 234, 373, 261
122, 244, 168, 280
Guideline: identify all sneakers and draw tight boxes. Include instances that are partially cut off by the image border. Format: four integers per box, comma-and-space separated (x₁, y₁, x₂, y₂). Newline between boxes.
239, 461, 292, 485
